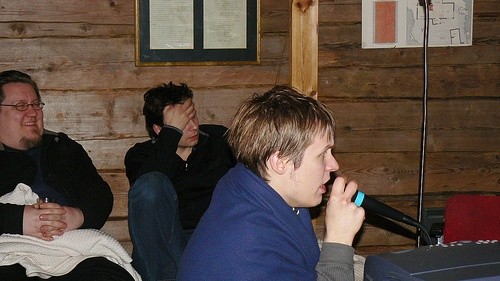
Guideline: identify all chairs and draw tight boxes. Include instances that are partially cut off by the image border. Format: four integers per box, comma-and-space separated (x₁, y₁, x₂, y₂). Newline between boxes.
443, 195, 500, 243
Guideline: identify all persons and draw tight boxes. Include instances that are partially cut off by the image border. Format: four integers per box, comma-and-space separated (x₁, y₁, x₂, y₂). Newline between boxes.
124, 81, 242, 281
0, 70, 136, 281
175, 85, 365, 281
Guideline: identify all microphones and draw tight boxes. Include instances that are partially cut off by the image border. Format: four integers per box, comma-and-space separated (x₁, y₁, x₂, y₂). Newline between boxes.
344, 184, 425, 230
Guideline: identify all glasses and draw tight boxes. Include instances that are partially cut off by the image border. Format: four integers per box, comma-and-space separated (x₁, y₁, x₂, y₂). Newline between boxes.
0, 101, 45, 111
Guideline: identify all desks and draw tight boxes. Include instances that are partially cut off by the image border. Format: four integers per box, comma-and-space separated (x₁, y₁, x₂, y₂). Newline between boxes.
365, 242, 500, 281
